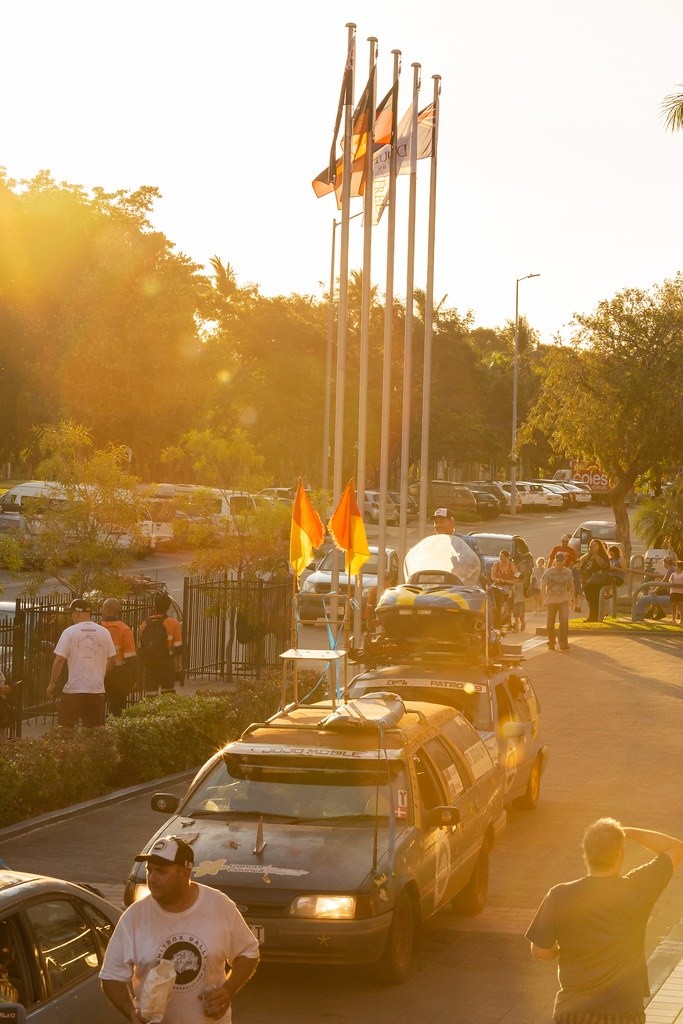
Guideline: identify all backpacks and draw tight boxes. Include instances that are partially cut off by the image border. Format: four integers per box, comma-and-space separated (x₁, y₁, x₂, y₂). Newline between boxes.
141, 616, 169, 665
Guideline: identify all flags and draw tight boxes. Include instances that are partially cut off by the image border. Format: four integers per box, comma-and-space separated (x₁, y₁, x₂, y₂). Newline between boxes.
290, 477, 325, 590
327, 477, 371, 576
312, 35, 437, 227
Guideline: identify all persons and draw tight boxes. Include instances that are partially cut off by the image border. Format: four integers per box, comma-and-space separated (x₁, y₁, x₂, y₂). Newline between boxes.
95, 598, 136, 717
580, 539, 611, 622
524, 818, 683, 1024
669, 561, 683, 625
605, 545, 627, 598
491, 550, 518, 630
99, 836, 260, 1024
531, 557, 547, 615
431, 508, 486, 590
547, 535, 577, 570
0, 671, 23, 741
138, 593, 183, 698
540, 553, 575, 650
644, 555, 677, 620
499, 573, 525, 632
46, 599, 117, 728
572, 559, 584, 612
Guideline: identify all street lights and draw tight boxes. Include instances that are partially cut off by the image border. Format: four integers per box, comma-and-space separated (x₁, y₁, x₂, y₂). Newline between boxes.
510, 273, 542, 515
321, 212, 367, 532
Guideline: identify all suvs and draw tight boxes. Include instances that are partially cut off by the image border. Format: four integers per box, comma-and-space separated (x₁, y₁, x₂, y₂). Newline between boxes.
124, 695, 508, 988
296, 546, 398, 627
336, 659, 550, 809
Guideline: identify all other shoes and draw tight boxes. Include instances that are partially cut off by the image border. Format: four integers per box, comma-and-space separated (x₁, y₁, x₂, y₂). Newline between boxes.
650, 612, 666, 619
562, 645, 570, 650
549, 645, 554, 650
583, 617, 599, 623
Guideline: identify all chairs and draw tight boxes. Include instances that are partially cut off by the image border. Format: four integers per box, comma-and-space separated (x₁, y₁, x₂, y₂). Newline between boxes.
274, 593, 349, 713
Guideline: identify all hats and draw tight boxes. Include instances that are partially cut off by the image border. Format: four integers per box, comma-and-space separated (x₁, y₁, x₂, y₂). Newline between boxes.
63, 599, 91, 612
555, 553, 564, 562
134, 836, 194, 867
660, 556, 673, 563
510, 572, 523, 580
429, 508, 453, 519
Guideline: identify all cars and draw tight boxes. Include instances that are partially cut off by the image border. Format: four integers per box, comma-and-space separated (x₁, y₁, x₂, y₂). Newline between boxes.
0, 865, 137, 1024
566, 520, 620, 557
0, 468, 673, 683
466, 530, 534, 591
